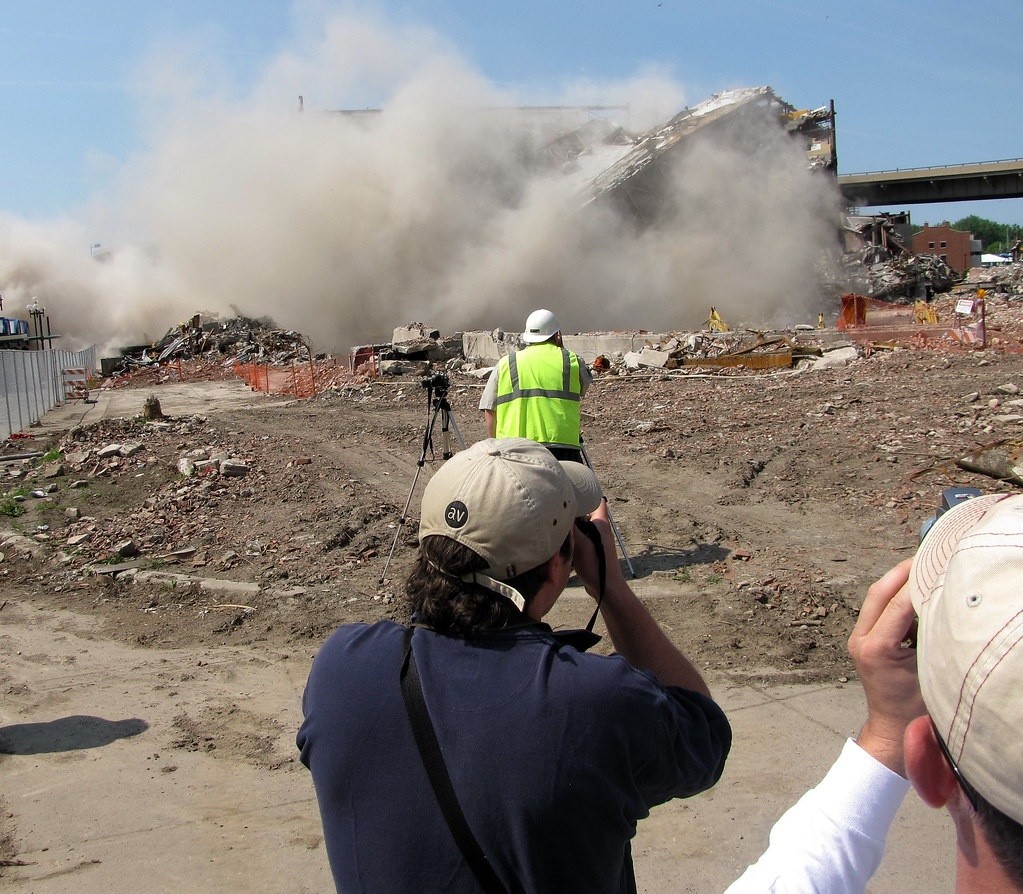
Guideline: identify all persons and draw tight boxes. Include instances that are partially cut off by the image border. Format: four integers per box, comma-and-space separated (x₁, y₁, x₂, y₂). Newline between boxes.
725, 492, 1023, 894
477, 309, 595, 465
294, 435, 733, 893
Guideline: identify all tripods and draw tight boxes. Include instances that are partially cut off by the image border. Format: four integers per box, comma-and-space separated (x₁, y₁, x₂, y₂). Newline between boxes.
377, 388, 466, 584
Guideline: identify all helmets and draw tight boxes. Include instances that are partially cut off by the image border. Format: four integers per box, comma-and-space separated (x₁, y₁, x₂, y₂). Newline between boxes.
522, 309, 561, 343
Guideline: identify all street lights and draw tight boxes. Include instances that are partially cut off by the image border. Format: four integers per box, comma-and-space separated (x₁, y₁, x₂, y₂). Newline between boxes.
25, 296, 39, 350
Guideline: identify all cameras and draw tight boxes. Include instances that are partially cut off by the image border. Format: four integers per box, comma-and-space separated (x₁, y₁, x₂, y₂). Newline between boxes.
421, 373, 450, 389
918, 486, 984, 547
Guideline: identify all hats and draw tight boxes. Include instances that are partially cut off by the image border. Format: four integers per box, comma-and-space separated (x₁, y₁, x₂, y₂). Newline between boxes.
908, 490, 1023, 826
418, 436, 601, 611
